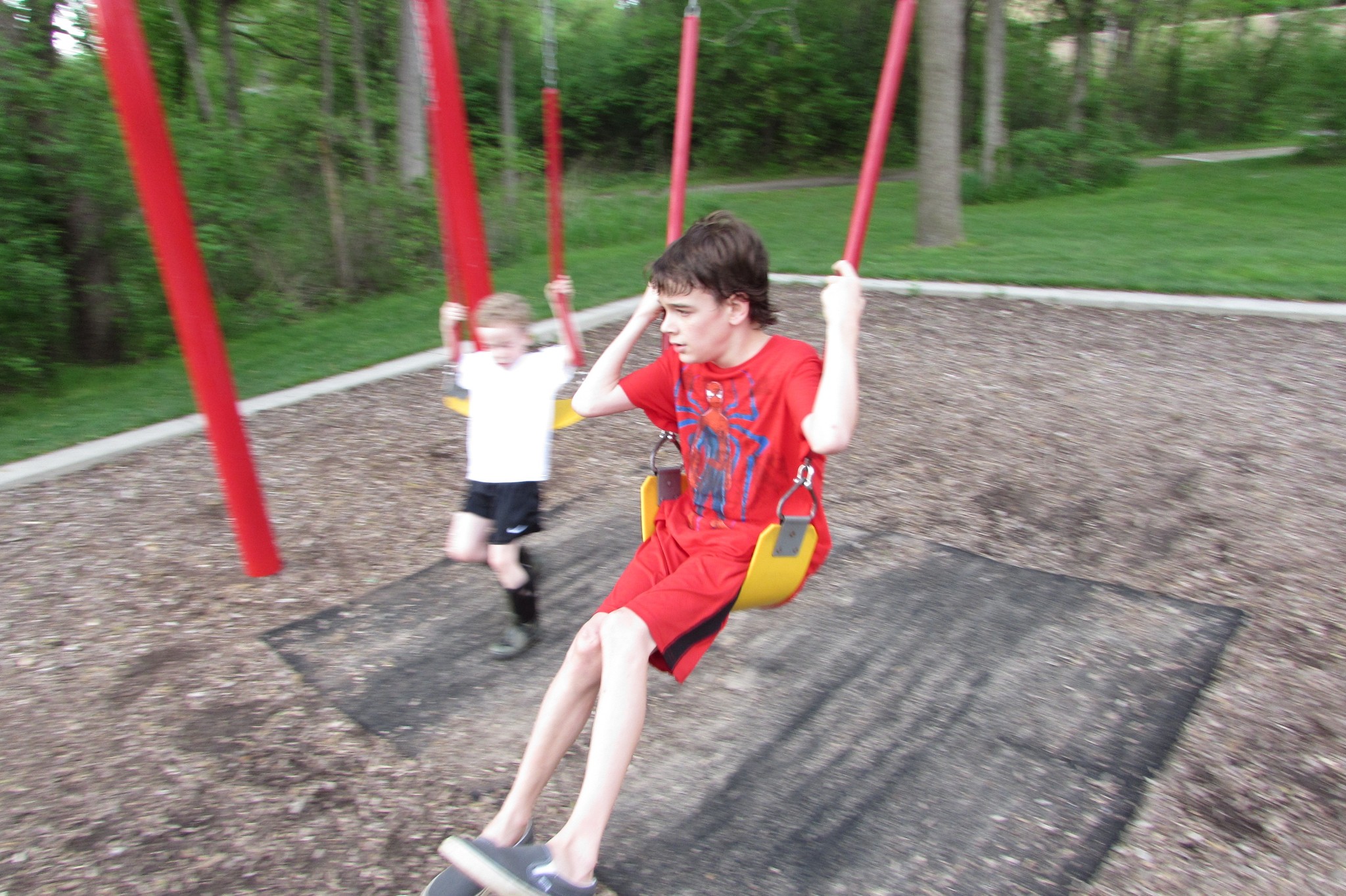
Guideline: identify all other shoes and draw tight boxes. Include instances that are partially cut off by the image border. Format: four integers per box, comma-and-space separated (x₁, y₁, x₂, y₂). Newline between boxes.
420, 823, 535, 896
436, 835, 598, 896
515, 546, 535, 579
488, 620, 538, 657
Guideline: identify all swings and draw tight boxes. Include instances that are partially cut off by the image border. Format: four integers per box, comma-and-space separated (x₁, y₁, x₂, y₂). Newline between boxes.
430, 0, 583, 431
628, 1, 919, 616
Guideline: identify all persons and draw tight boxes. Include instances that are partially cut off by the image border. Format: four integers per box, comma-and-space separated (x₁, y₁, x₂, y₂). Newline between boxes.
440, 272, 587, 658
421, 209, 868, 895
